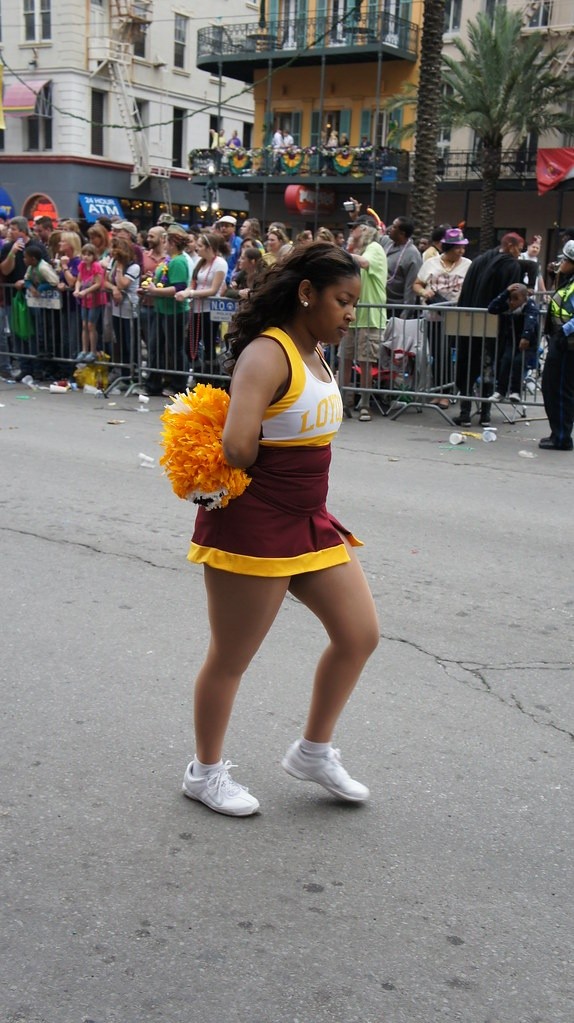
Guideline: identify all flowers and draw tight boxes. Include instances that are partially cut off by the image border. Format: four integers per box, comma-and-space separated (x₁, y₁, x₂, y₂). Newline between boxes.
158, 381, 250, 511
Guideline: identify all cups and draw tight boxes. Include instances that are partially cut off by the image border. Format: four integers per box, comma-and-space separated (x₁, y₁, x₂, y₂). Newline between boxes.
482, 431, 497, 442
449, 432, 467, 445
83, 384, 98, 395
49, 384, 67, 393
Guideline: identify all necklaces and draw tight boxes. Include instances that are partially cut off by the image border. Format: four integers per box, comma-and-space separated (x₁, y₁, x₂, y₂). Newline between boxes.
383, 239, 410, 283
141, 256, 170, 289
440, 260, 456, 272
189, 254, 217, 358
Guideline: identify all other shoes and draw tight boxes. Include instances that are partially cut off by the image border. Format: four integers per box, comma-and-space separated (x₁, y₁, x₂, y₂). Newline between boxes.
131, 387, 150, 395
539, 438, 573, 450
452, 413, 471, 426
489, 392, 503, 402
162, 388, 174, 397
479, 412, 490, 426
430, 398, 449, 408
76, 351, 96, 362
509, 393, 520, 402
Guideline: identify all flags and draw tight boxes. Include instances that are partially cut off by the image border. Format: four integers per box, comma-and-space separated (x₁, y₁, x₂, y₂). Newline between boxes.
536, 147, 574, 196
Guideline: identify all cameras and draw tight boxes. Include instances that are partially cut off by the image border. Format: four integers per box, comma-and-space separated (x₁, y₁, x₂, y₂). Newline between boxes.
343, 201, 354, 211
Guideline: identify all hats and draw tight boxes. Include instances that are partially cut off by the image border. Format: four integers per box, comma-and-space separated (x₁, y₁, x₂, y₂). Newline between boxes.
215, 216, 237, 226
157, 213, 175, 225
111, 222, 138, 238
440, 228, 468, 245
557, 239, 574, 261
347, 214, 376, 229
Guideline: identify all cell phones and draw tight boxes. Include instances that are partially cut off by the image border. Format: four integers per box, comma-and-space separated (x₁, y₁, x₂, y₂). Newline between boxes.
17, 238, 23, 243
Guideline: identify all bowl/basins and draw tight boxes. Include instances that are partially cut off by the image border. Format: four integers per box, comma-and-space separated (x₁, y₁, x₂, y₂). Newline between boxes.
343, 201, 355, 211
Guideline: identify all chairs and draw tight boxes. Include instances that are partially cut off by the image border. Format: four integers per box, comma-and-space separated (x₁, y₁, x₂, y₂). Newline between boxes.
222, 27, 243, 54
273, 27, 288, 51
351, 317, 434, 417
374, 13, 390, 45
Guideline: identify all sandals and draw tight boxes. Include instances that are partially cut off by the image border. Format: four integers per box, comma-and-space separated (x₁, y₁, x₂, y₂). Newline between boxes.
359, 406, 371, 420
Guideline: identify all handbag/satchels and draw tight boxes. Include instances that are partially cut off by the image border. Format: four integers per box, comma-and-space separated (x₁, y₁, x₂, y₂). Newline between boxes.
425, 292, 452, 316
10, 290, 34, 339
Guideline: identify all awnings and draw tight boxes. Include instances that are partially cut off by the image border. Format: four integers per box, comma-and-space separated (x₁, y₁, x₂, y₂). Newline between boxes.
3, 80, 53, 119
77, 195, 126, 223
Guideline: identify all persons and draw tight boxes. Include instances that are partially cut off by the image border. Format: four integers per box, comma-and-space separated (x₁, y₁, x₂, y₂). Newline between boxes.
270, 128, 294, 175
0, 195, 574, 449
210, 128, 226, 148
181, 241, 381, 813
226, 130, 240, 146
321, 131, 372, 176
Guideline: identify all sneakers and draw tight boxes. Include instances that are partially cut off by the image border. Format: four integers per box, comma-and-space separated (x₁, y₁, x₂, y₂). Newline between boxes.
182, 761, 259, 816
282, 741, 370, 802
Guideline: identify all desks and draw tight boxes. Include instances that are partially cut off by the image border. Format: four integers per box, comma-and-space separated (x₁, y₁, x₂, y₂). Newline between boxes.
247, 34, 276, 54
343, 27, 374, 47
